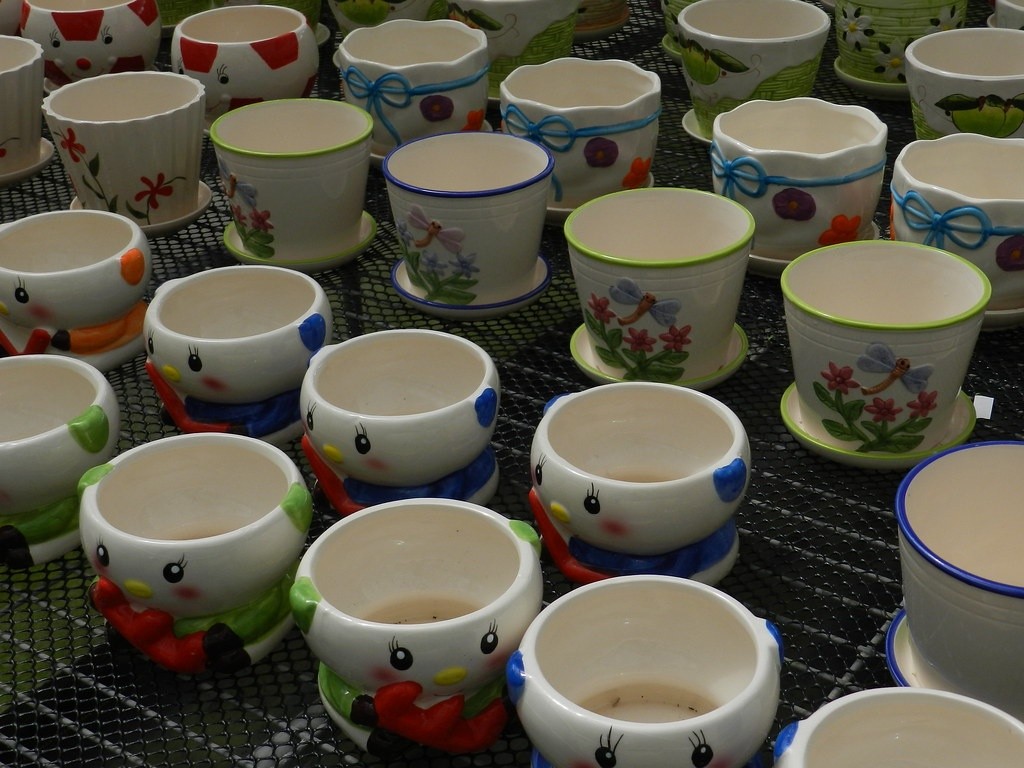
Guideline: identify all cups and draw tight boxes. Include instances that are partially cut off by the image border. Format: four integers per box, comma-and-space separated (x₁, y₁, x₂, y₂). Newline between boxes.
0, 1, 1024, 767
